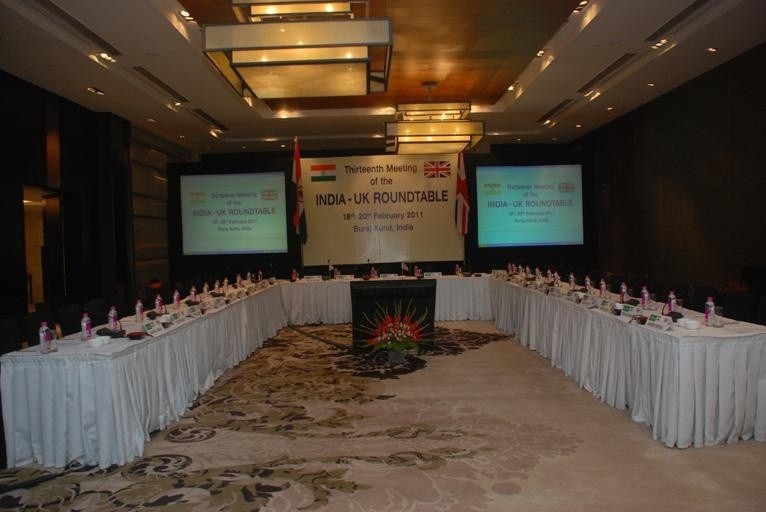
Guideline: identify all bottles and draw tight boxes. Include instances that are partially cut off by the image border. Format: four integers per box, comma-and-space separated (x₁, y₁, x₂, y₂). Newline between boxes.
668, 291, 676, 312
81, 313, 91, 339
371, 267, 375, 278
585, 274, 649, 307
109, 306, 117, 329
39, 321, 51, 353
705, 296, 715, 326
190, 269, 262, 300
508, 263, 573, 291
135, 289, 180, 320
455, 264, 460, 276
292, 269, 296, 279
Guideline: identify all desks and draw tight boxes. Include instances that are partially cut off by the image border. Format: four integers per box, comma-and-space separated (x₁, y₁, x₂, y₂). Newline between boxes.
286, 264, 497, 325
498, 267, 764, 446
1, 273, 281, 471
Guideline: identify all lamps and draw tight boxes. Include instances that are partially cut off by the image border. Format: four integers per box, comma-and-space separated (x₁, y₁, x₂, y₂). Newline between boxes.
380, 81, 484, 156
199, 1, 392, 99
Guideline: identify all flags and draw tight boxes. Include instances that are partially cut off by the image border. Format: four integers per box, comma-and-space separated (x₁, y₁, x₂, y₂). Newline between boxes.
455, 152, 470, 236
291, 139, 308, 244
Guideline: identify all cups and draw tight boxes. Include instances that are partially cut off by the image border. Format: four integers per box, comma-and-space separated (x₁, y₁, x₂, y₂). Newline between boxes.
716, 307, 722, 325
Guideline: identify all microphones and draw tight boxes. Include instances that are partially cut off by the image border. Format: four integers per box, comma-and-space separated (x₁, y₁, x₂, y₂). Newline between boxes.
179, 287, 203, 306
620, 289, 639, 306
82, 306, 126, 337
509, 268, 588, 293
208, 273, 260, 298
363, 259, 379, 280
321, 259, 335, 280
661, 290, 689, 322
138, 295, 169, 320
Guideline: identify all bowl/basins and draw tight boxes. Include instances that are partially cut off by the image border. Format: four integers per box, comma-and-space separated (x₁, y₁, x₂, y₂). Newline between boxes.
677, 319, 700, 331
88, 336, 111, 347
